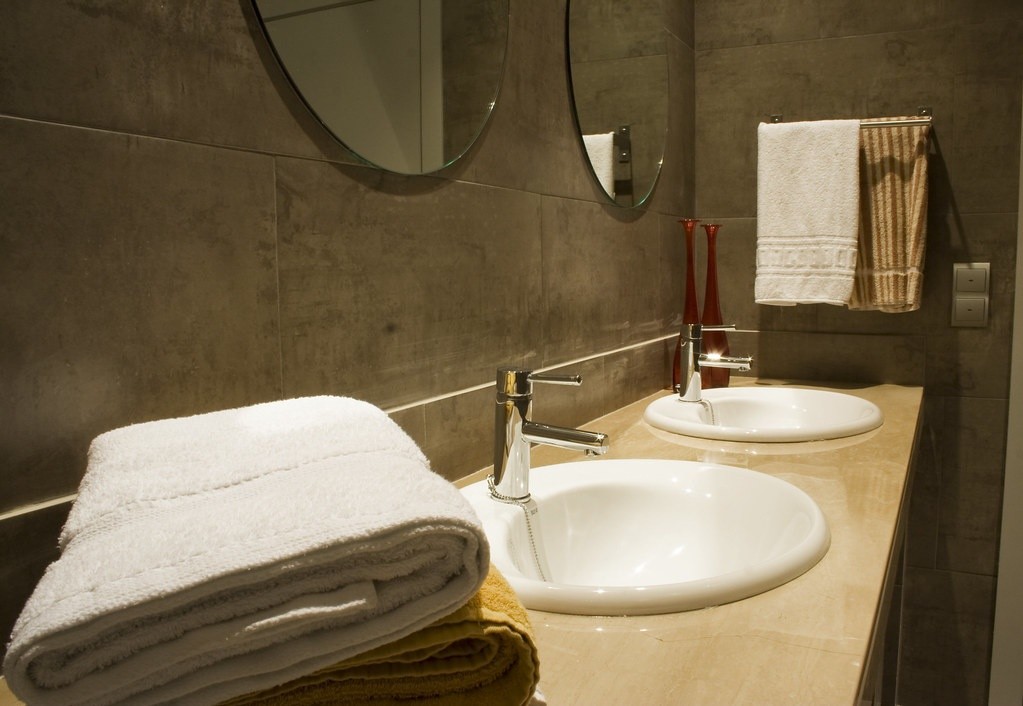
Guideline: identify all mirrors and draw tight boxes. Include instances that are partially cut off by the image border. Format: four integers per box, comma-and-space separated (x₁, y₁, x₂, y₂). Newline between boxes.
565, 0, 670, 209
240, 0, 510, 176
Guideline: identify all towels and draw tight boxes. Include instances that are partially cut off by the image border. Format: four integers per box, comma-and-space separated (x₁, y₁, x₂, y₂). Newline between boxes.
4, 395, 547, 706
754, 116, 932, 313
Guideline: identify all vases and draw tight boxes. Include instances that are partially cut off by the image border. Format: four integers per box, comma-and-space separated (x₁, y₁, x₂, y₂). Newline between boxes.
672, 219, 702, 392
698, 224, 729, 389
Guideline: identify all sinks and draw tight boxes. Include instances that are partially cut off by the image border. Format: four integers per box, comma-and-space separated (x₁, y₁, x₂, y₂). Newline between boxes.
639, 417, 884, 455
458, 459, 831, 616
644, 388, 884, 443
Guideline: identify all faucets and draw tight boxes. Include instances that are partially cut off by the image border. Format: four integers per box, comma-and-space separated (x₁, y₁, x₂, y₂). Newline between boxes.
679, 323, 752, 402
490, 367, 608, 505
